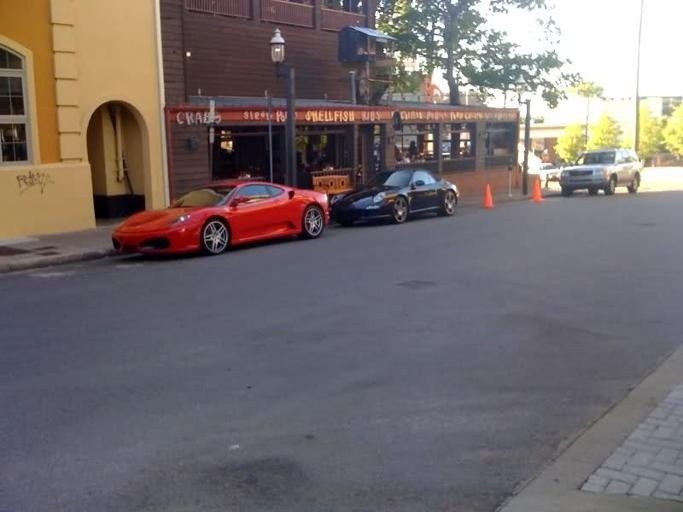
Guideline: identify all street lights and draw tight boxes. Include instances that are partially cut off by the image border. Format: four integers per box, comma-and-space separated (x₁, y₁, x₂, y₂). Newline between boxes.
269, 28, 298, 186
514, 73, 531, 193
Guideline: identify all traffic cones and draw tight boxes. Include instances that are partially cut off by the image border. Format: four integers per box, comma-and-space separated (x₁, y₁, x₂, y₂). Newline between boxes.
530, 180, 547, 202
483, 184, 494, 208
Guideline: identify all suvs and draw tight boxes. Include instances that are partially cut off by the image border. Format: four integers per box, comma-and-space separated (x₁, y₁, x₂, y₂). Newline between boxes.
558, 149, 641, 197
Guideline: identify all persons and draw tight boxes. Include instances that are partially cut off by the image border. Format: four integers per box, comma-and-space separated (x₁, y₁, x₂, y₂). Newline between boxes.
461, 141, 469, 152
238, 170, 251, 179
297, 159, 310, 188
255, 155, 269, 182
395, 145, 400, 157
406, 141, 419, 161
319, 152, 329, 170
